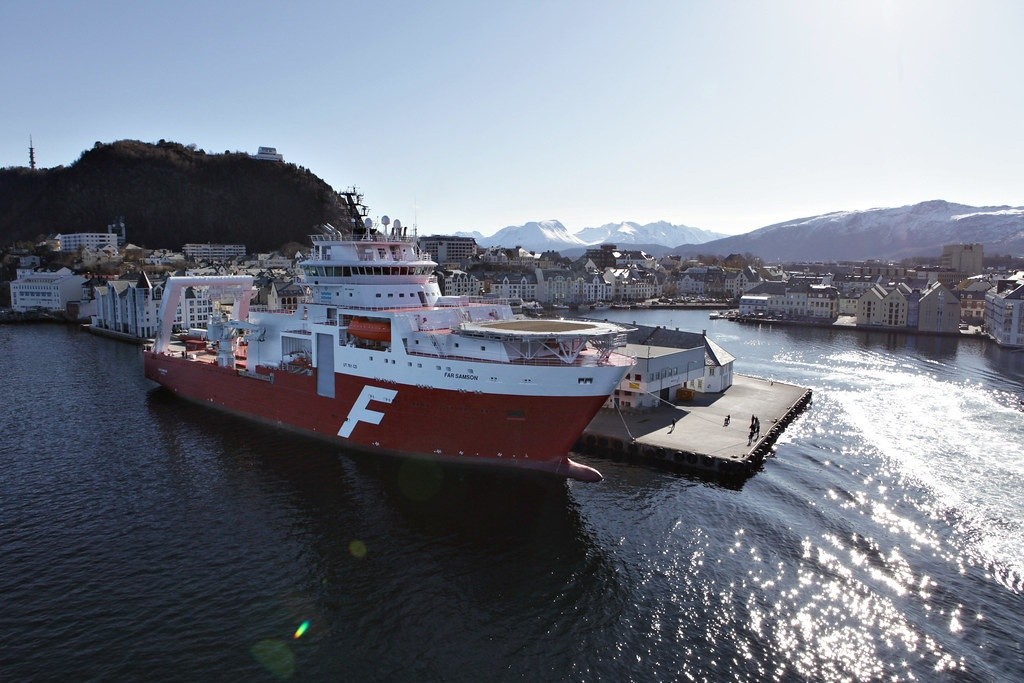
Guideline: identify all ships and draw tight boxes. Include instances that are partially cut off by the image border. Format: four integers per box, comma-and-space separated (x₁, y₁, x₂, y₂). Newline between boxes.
135, 235, 639, 486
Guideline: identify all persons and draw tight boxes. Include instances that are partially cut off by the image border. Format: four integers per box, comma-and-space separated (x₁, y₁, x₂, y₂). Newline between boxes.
747, 414, 762, 446
672, 418, 677, 428
726, 414, 731, 424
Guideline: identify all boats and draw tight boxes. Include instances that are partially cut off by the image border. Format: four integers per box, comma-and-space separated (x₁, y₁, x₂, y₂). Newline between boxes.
709, 310, 721, 319
590, 302, 607, 311
611, 303, 632, 310
